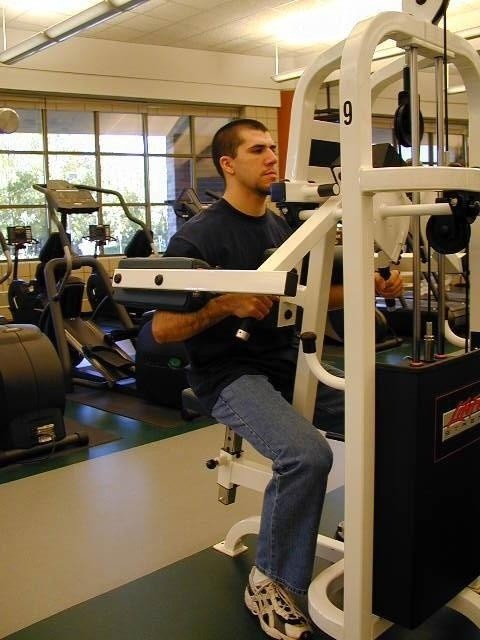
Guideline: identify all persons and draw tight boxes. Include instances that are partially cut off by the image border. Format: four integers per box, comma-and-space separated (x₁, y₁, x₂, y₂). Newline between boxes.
151, 118, 405, 639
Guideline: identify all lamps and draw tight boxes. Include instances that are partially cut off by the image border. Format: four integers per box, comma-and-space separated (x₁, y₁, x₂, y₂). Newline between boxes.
0, 1, 151, 67
270, 26, 480, 84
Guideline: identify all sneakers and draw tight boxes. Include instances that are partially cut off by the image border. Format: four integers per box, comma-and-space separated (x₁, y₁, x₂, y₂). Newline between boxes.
244, 566, 313, 640
